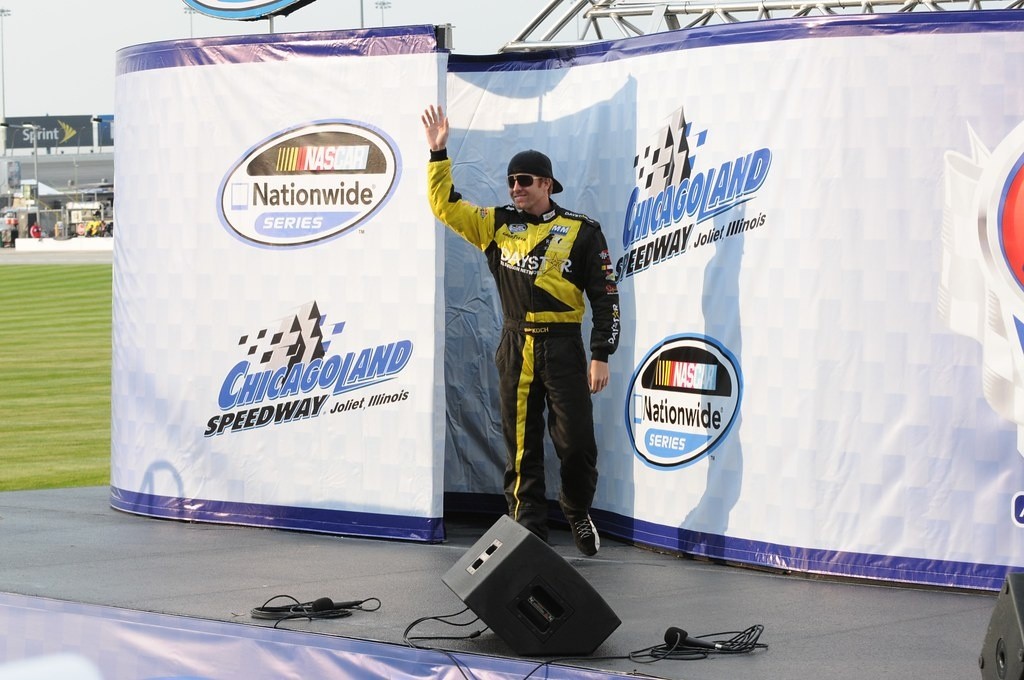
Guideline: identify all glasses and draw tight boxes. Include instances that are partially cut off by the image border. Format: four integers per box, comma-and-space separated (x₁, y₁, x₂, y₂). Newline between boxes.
508, 175, 547, 188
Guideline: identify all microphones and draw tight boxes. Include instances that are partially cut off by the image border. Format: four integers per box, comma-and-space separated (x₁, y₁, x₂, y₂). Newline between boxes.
311, 597, 365, 612
664, 626, 732, 652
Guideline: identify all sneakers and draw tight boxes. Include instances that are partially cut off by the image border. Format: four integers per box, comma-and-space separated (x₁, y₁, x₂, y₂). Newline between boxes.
570, 513, 600, 556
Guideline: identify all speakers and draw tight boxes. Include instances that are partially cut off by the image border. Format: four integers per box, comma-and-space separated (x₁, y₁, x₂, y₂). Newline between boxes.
977, 569, 1024, 680
442, 515, 623, 655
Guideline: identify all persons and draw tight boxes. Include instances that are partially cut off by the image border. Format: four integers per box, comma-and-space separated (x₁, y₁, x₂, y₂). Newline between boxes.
86, 220, 113, 237
420, 104, 621, 557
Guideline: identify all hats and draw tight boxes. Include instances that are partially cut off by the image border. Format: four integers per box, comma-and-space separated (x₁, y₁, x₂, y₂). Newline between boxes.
507, 149, 563, 194
